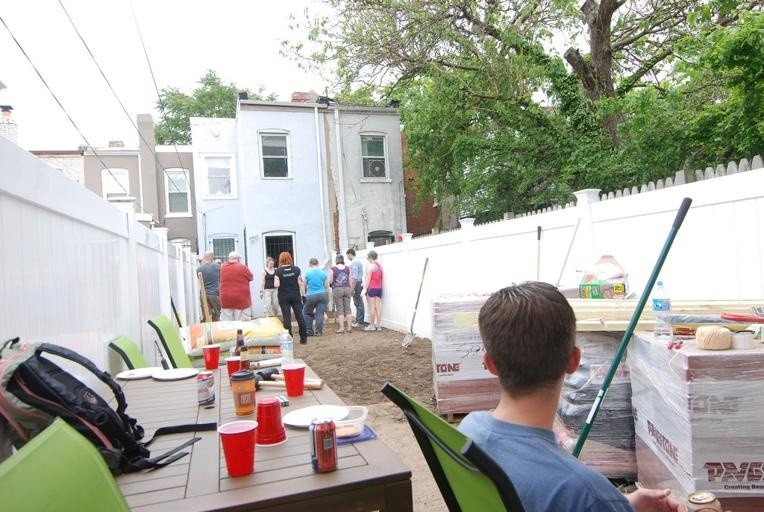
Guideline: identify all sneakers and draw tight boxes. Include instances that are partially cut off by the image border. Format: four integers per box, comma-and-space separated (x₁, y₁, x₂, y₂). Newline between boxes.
336, 322, 381, 333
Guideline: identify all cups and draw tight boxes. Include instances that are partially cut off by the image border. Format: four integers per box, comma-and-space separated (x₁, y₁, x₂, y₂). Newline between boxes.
217, 420, 259, 477
202, 345, 220, 370
230, 369, 258, 418
225, 355, 240, 379
253, 396, 288, 446
281, 363, 307, 398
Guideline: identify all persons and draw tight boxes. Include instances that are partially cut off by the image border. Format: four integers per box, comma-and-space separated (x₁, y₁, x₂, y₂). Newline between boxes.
346, 249, 365, 328
273, 252, 308, 344
259, 257, 282, 318
456, 280, 680, 512
301, 257, 329, 336
328, 253, 353, 334
360, 250, 382, 331
195, 248, 254, 321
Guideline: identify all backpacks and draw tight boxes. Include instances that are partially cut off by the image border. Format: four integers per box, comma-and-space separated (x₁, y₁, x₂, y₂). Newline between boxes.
0, 336, 217, 475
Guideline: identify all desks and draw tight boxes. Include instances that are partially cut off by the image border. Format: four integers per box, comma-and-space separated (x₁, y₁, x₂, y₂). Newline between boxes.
83, 358, 415, 512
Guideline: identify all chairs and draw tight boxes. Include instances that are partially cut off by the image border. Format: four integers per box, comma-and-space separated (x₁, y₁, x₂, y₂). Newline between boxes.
107, 334, 148, 373
376, 382, 524, 511
145, 313, 196, 369
0, 413, 133, 512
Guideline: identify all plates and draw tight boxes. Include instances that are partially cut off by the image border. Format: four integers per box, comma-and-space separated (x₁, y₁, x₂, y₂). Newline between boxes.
152, 368, 198, 381
283, 406, 350, 429
116, 366, 164, 380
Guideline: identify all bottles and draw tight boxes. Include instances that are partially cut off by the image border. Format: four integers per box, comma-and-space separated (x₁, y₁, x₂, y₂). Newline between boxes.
233, 329, 245, 354
652, 280, 673, 342
280, 329, 294, 365
239, 347, 252, 370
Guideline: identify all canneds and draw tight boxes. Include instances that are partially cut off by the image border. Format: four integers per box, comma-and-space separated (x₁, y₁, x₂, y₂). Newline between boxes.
310, 418, 337, 474
197, 370, 214, 405
687, 493, 722, 511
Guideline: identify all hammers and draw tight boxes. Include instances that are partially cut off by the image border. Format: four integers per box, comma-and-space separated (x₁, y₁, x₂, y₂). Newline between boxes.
257, 368, 325, 385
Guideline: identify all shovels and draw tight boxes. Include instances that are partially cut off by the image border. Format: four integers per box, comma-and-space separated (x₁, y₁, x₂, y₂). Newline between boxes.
402, 258, 429, 347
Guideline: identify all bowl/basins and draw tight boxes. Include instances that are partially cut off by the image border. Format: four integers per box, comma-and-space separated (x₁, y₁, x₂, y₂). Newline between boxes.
731, 333, 755, 350
